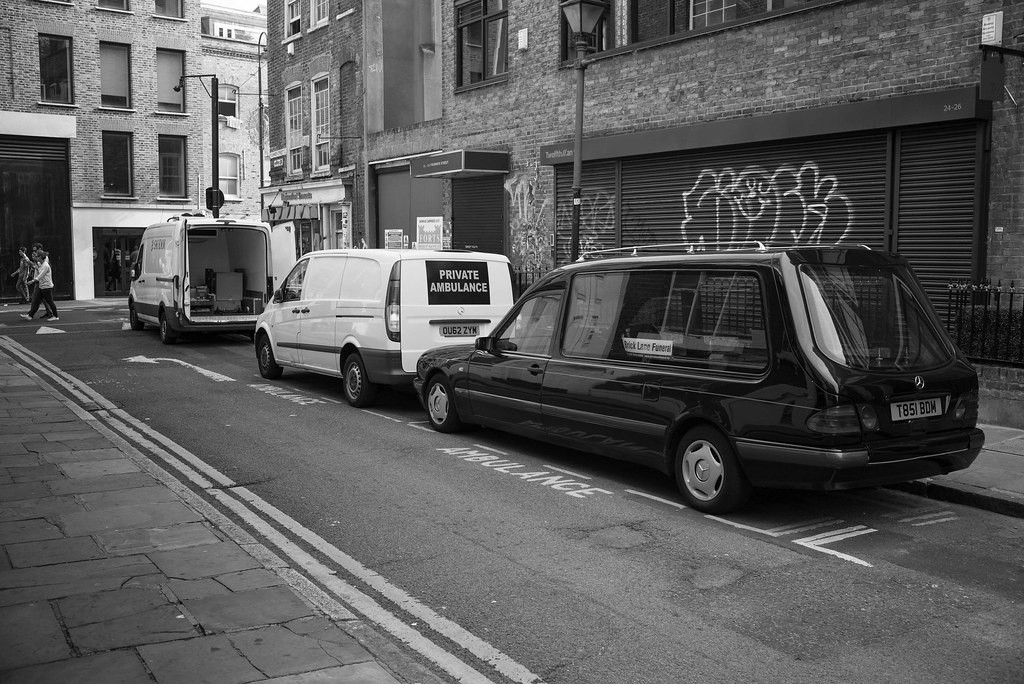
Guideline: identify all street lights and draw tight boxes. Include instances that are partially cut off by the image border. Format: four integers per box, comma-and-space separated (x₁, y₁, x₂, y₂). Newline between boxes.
559, 0, 611, 263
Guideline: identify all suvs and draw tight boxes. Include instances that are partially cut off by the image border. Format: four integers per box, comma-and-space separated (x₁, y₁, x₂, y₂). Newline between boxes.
414, 240, 987, 514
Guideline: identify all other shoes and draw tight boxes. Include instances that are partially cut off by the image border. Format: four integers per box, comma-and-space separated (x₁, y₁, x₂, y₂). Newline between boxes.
39, 314, 53, 319
19, 300, 30, 305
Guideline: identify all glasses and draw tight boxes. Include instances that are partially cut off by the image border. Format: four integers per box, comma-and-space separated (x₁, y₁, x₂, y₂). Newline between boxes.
32, 249, 38, 253
35, 256, 39, 258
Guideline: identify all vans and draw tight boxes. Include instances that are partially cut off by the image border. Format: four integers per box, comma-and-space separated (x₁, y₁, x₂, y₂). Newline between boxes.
126, 214, 296, 346
253, 248, 522, 408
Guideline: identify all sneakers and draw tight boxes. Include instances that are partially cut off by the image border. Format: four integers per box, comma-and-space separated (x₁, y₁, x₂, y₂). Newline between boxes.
19, 313, 33, 321
46, 315, 59, 322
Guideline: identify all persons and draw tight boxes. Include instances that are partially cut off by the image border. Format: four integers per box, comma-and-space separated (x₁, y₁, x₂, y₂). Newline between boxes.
11, 242, 59, 321
94, 241, 139, 291
